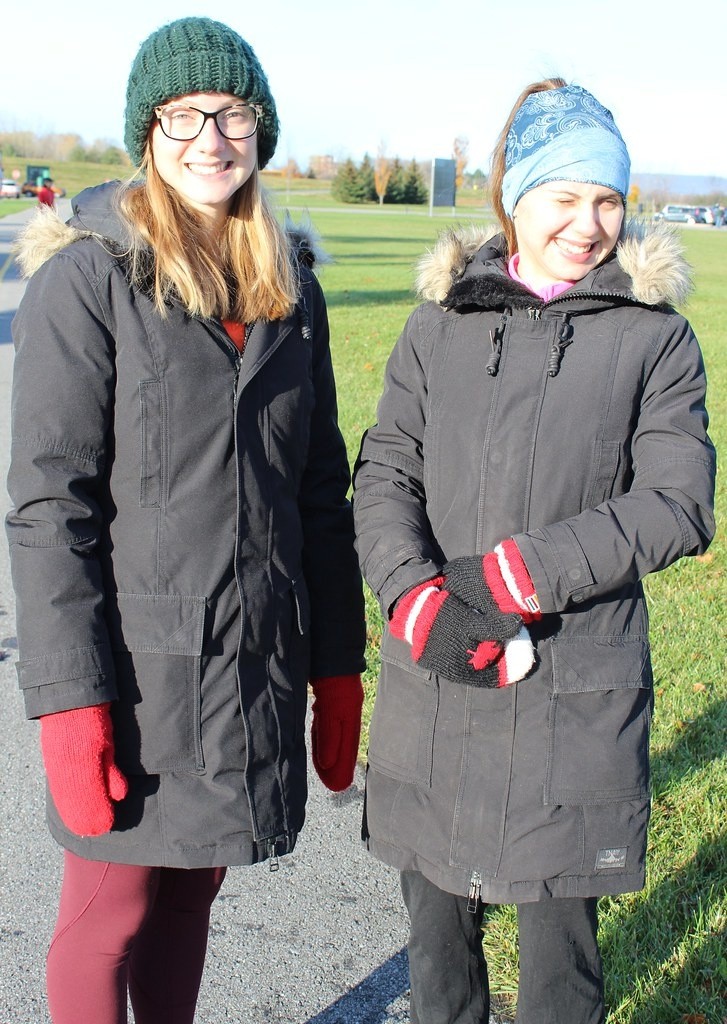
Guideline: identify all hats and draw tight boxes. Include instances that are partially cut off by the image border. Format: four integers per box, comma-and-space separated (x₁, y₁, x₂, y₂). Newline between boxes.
124, 18, 278, 172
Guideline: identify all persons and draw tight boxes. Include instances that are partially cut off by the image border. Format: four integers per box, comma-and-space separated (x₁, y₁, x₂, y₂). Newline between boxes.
4, 18, 365, 1024
38, 177, 59, 208
695, 206, 727, 229
354, 78, 716, 1024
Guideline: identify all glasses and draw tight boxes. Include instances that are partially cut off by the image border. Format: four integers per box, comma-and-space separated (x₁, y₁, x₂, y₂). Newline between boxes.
150, 101, 263, 141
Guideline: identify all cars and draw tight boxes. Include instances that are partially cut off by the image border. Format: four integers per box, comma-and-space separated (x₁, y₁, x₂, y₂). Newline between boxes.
21, 181, 66, 198
1, 179, 20, 199
654, 203, 716, 225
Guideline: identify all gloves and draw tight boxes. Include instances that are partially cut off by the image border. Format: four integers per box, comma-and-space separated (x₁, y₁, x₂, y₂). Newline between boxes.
41, 705, 127, 837
439, 537, 542, 627
390, 578, 536, 688
312, 674, 364, 791
